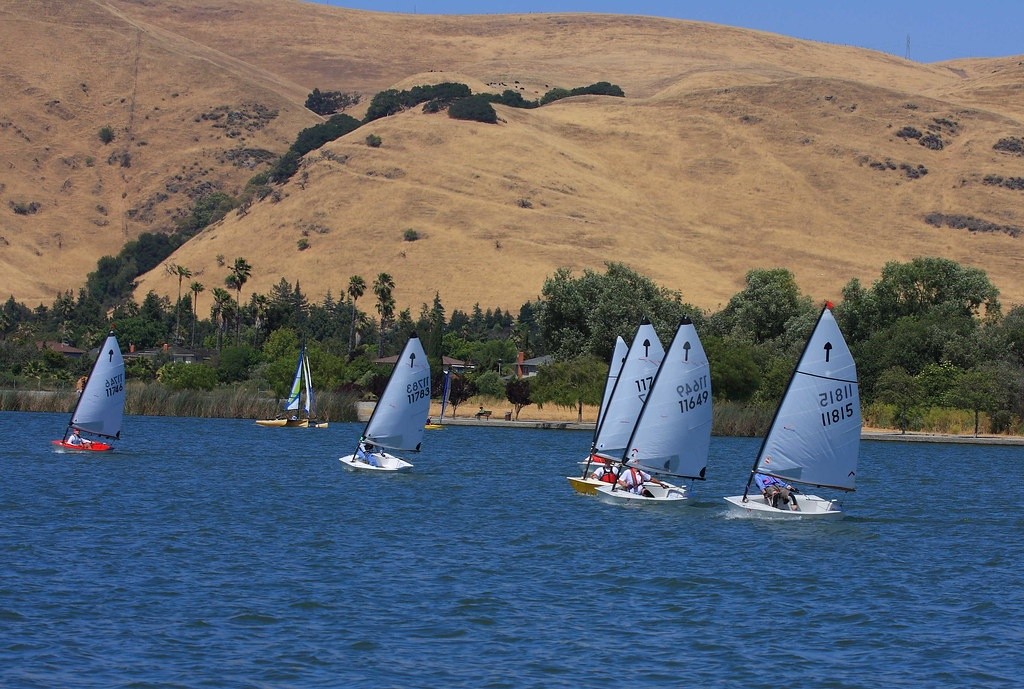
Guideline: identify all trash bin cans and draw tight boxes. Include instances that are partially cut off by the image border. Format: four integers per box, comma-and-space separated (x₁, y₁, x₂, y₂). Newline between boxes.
505, 412, 511, 421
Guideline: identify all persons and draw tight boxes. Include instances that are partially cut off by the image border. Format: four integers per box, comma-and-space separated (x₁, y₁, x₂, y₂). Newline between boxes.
477, 407, 484, 419
585, 453, 605, 463
362, 444, 377, 466
618, 467, 669, 498
67, 429, 83, 445
425, 416, 432, 425
590, 459, 621, 484
755, 473, 801, 511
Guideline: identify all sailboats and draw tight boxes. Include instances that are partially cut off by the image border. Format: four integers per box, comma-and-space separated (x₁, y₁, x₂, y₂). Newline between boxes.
722, 301, 863, 523
253, 334, 329, 429
566, 313, 713, 509
51, 322, 126, 453
338, 330, 431, 473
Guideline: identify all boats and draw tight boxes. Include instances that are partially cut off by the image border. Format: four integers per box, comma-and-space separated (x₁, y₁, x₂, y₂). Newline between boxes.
425, 424, 442, 429
